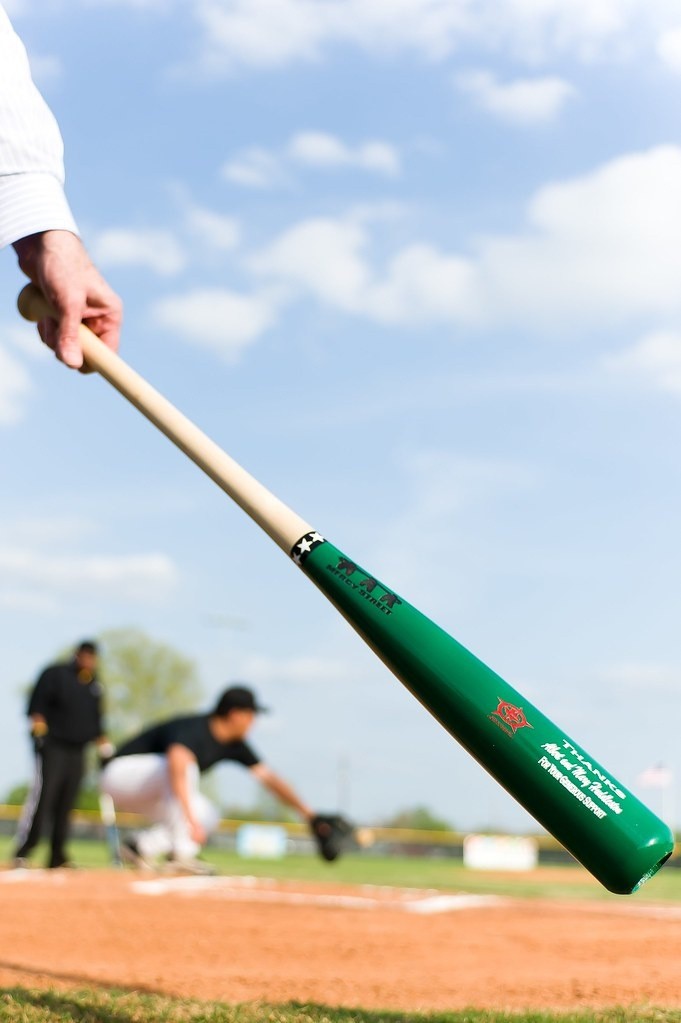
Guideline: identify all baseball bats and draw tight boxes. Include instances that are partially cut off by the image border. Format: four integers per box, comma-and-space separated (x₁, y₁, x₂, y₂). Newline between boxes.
78, 321, 677, 894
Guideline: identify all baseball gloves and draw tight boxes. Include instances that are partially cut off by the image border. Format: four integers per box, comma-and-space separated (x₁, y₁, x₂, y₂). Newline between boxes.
310, 815, 357, 867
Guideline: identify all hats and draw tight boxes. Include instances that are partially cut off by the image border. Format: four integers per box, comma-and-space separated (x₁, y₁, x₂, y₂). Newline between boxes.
225, 689, 266, 713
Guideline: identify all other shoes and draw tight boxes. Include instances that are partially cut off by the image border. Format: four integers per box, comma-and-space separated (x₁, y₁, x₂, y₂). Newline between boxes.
119, 836, 153, 872
166, 855, 213, 875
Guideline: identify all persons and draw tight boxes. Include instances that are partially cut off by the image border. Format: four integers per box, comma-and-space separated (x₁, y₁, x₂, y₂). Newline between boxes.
7, 640, 117, 871
97, 686, 354, 882
0, 6, 123, 378
